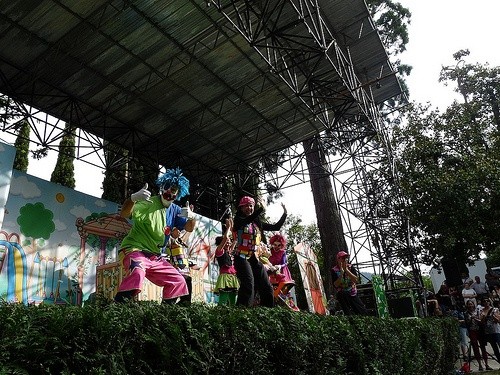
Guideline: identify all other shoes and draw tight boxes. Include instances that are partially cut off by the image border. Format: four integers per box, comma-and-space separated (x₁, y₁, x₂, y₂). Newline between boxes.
486, 366, 493, 370
479, 366, 483, 371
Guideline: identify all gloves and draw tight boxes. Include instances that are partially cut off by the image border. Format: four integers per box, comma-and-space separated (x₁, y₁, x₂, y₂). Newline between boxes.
178, 200, 195, 220
131, 183, 154, 203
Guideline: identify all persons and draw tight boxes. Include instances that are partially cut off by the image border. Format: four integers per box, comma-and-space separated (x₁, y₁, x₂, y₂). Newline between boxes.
464, 300, 493, 370
480, 296, 500, 364
470, 276, 490, 299
215, 217, 241, 308
331, 251, 363, 315
461, 283, 477, 308
492, 285, 500, 300
326, 295, 336, 315
233, 196, 287, 309
164, 224, 193, 306
116, 168, 196, 306
415, 285, 459, 316
269, 234, 291, 284
485, 266, 500, 293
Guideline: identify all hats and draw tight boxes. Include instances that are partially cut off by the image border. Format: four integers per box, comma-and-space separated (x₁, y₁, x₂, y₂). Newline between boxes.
337, 251, 350, 259
238, 196, 255, 206
